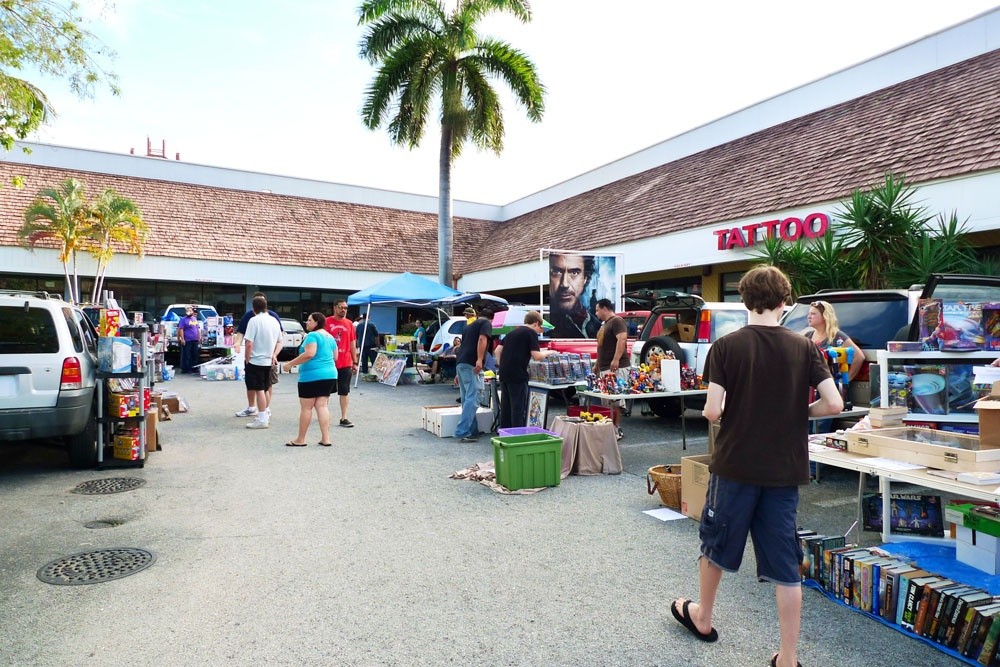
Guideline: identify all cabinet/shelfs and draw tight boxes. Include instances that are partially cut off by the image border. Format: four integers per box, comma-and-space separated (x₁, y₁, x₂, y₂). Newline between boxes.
93, 322, 151, 474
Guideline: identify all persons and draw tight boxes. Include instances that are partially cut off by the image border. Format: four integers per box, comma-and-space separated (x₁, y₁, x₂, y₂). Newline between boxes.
549, 252, 604, 338
670, 266, 844, 667
352, 312, 381, 374
590, 298, 631, 441
175, 305, 201, 375
321, 298, 358, 428
462, 306, 478, 325
453, 307, 494, 440
245, 298, 284, 429
413, 319, 428, 349
452, 349, 495, 404
801, 299, 865, 482
424, 335, 462, 384
283, 311, 339, 447
494, 311, 557, 428
232, 291, 283, 417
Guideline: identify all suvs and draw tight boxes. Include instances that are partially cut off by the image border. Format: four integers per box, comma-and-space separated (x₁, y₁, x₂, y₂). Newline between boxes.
0, 288, 99, 468
779, 284, 1000, 432
619, 290, 791, 420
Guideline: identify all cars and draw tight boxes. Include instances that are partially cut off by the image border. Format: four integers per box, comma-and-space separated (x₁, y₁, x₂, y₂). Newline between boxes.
126, 310, 160, 335
161, 304, 227, 358
278, 318, 308, 357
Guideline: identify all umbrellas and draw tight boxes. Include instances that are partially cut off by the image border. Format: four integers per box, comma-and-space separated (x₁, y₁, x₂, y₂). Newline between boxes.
490, 307, 556, 338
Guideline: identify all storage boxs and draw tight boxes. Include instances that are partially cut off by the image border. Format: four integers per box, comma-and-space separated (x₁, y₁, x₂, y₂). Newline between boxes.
490, 426, 564, 488
679, 454, 714, 524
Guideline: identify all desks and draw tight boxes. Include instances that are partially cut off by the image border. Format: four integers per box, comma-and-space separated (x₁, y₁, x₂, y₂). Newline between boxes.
809, 404, 873, 484
806, 433, 1000, 553
373, 348, 430, 383
579, 386, 709, 449
490, 370, 588, 431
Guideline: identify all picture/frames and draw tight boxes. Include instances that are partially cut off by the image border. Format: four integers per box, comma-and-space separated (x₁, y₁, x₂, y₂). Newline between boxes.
525, 387, 551, 429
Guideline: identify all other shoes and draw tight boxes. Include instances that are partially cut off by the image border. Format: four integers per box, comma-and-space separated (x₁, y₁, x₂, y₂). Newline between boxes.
181, 368, 200, 373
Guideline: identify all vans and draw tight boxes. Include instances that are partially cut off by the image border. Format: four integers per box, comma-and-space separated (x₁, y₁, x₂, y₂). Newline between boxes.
426, 307, 469, 377
432, 293, 551, 340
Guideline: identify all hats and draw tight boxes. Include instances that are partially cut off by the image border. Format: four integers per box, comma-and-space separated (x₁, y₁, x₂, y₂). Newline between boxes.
192, 308, 198, 313
462, 307, 475, 315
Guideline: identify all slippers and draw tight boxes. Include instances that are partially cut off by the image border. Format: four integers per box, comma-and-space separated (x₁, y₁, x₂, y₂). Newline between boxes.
318, 440, 332, 446
771, 653, 802, 667
286, 441, 307, 446
671, 599, 718, 642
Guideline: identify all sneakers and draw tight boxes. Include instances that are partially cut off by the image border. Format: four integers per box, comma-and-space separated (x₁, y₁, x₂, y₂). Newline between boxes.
236, 406, 257, 416
268, 409, 272, 416
340, 417, 354, 427
615, 426, 624, 441
245, 416, 270, 429
462, 435, 479, 441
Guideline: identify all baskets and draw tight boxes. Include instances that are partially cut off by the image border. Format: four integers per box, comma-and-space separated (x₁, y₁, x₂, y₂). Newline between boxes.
646, 463, 681, 508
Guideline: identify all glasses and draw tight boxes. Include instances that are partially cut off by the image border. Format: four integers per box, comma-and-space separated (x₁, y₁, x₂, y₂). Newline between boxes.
335, 305, 348, 310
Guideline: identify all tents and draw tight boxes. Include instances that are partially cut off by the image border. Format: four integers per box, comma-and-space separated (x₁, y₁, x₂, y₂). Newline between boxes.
344, 271, 464, 388
432, 290, 509, 329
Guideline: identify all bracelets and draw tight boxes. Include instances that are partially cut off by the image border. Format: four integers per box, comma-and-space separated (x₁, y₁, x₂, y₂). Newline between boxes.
352, 361, 358, 365
288, 362, 292, 367
234, 343, 240, 348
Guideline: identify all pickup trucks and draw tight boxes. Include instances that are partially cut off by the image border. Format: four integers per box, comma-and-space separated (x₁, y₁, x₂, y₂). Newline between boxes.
537, 309, 679, 401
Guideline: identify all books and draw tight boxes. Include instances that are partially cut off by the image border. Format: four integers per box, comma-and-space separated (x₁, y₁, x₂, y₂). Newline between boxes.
796, 528, 1000, 667
